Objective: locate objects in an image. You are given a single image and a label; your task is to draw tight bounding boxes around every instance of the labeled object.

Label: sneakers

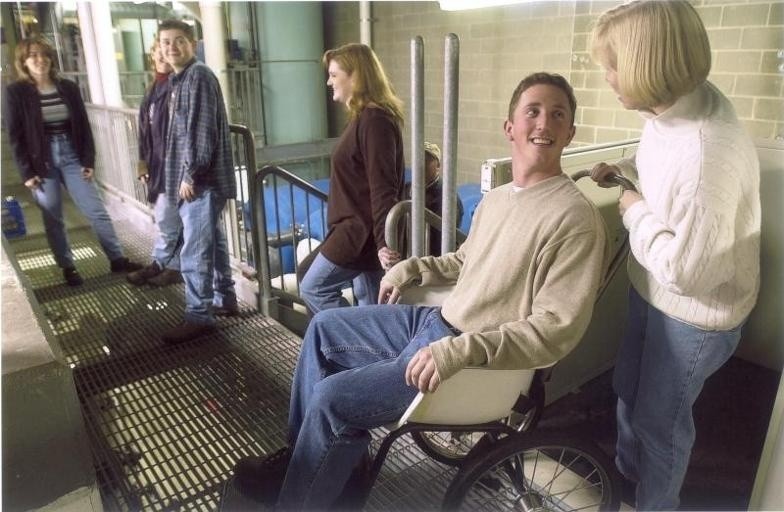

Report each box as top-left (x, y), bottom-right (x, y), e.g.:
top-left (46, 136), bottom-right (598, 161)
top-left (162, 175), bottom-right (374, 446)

top-left (166, 307), bottom-right (233, 343)
top-left (110, 257), bottom-right (179, 286)
top-left (63, 268), bottom-right (83, 286)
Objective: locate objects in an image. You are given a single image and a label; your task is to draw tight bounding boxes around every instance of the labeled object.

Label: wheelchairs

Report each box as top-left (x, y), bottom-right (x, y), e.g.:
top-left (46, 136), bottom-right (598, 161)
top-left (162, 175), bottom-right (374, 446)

top-left (215, 169), bottom-right (638, 512)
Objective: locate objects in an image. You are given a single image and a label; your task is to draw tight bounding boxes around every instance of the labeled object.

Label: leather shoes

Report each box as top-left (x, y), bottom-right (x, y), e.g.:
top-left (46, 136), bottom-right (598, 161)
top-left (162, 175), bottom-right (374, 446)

top-left (232, 446), bottom-right (293, 486)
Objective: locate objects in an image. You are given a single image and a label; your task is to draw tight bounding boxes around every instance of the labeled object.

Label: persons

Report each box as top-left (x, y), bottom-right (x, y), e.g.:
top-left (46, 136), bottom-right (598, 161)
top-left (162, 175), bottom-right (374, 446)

top-left (577, 1), bottom-right (763, 509)
top-left (406, 142), bottom-right (465, 253)
top-left (295, 40), bottom-right (404, 316)
top-left (150, 13), bottom-right (241, 347)
top-left (232, 72), bottom-right (611, 509)
top-left (5, 35), bottom-right (148, 288)
top-left (124, 38), bottom-right (187, 286)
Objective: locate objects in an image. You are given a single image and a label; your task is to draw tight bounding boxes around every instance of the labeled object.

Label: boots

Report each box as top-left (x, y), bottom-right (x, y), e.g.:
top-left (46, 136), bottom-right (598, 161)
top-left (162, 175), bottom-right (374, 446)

top-left (425, 142), bottom-right (440, 161)
